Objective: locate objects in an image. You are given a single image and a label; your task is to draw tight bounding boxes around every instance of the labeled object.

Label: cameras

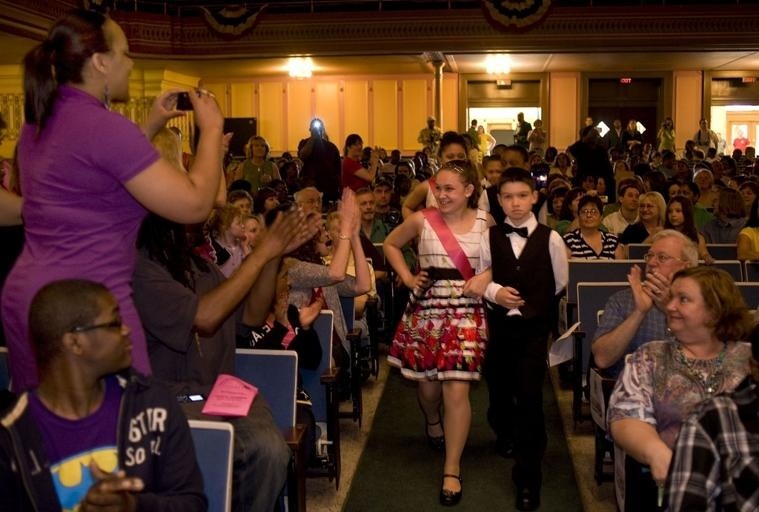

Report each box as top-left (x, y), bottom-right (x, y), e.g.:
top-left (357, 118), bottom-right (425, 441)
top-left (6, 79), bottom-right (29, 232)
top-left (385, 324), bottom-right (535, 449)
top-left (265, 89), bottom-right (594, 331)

top-left (177, 92), bottom-right (194, 110)
top-left (383, 209), bottom-right (401, 225)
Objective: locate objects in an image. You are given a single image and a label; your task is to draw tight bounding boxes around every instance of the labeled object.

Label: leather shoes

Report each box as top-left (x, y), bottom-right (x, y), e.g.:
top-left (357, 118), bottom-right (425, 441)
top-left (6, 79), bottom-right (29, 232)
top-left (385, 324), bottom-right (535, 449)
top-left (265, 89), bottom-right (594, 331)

top-left (440, 474), bottom-right (463, 506)
top-left (426, 418), bottom-right (445, 449)
top-left (516, 490), bottom-right (540, 510)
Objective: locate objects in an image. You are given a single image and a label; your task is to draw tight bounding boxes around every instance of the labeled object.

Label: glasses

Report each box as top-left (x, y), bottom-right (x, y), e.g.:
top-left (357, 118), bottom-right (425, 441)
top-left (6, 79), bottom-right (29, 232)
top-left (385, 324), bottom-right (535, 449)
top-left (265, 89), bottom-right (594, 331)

top-left (644, 254), bottom-right (677, 262)
top-left (75, 318), bottom-right (122, 331)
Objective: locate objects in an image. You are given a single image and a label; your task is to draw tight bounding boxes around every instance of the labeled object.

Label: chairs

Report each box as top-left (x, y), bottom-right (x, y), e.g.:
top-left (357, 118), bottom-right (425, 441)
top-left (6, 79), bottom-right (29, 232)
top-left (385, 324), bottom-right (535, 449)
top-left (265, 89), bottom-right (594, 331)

top-left (558, 242), bottom-right (759, 512)
top-left (1, 346), bottom-right (11, 391)
top-left (187, 238), bottom-right (387, 512)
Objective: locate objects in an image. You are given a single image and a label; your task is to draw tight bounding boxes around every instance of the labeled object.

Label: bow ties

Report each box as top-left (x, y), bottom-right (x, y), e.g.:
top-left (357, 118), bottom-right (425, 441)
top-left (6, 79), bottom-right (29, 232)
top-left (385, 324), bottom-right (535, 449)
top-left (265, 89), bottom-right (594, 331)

top-left (504, 223), bottom-right (528, 237)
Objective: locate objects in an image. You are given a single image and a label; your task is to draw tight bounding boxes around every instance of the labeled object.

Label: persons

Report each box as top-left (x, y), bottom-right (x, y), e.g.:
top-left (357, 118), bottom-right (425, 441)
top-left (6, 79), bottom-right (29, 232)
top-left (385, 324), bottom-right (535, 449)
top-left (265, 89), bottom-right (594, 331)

top-left (592, 229), bottom-right (699, 370)
top-left (467, 119), bottom-right (496, 154)
top-left (660, 322), bottom-right (759, 512)
top-left (515, 112), bottom-right (546, 157)
top-left (129, 200), bottom-right (321, 512)
top-left (382, 127), bottom-right (569, 512)
top-left (606, 263), bottom-right (759, 488)
top-left (0, 9), bottom-right (225, 392)
top-left (0, 277), bottom-right (209, 512)
top-left (562, 116), bottom-right (759, 263)
top-left (152, 118), bottom-right (442, 385)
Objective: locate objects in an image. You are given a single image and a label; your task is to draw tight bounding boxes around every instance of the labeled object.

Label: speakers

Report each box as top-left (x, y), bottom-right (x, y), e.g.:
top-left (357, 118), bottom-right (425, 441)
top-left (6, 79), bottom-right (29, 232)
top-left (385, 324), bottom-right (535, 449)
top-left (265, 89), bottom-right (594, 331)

top-left (194, 117), bottom-right (257, 158)
